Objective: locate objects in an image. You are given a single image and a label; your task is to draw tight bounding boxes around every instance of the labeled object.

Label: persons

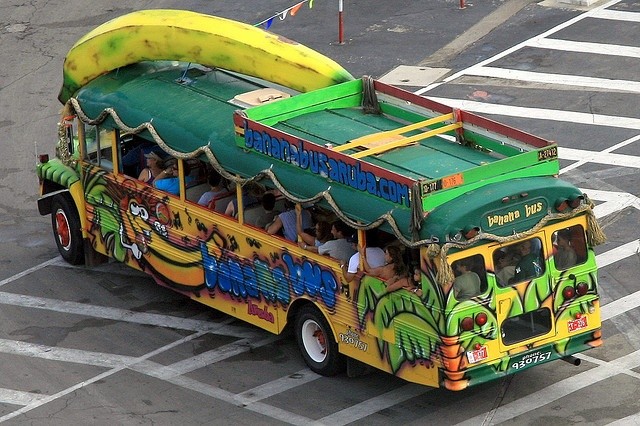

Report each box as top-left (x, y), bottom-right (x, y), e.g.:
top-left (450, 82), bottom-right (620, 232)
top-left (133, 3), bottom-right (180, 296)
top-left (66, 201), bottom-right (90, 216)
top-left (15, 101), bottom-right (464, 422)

top-left (357, 242), bottom-right (402, 281)
top-left (386, 263), bottom-right (413, 292)
top-left (255, 193), bottom-right (282, 228)
top-left (152, 158), bottom-right (195, 196)
top-left (297, 222), bottom-right (356, 264)
top-left (224, 184), bottom-right (259, 218)
top-left (266, 197), bottom-right (312, 242)
top-left (295, 206), bottom-right (334, 247)
top-left (121, 139), bottom-right (150, 178)
top-left (553, 229), bottom-right (578, 270)
top-left (412, 266), bottom-right (423, 297)
top-left (515, 241), bottom-right (544, 281)
top-left (340, 234), bottom-right (388, 283)
top-left (136, 150), bottom-right (164, 185)
top-left (196, 171), bottom-right (228, 206)
top-left (454, 259), bottom-right (482, 297)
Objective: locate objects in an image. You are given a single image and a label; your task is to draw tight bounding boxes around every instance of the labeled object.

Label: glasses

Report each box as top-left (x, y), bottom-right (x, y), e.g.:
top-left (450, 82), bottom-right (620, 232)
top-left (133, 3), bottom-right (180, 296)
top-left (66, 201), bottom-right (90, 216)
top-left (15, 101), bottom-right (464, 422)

top-left (173, 166), bottom-right (177, 170)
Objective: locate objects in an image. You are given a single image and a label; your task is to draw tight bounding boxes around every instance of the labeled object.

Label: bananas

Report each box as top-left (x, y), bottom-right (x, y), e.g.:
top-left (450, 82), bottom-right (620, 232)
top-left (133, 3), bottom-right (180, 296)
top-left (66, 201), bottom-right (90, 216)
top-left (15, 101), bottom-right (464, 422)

top-left (119, 194), bottom-right (224, 287)
top-left (59, 8), bottom-right (354, 106)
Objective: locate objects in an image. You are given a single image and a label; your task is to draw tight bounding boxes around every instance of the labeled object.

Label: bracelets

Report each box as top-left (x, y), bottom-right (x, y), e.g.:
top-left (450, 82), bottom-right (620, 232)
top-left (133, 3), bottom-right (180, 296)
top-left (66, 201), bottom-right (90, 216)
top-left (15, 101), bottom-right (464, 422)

top-left (162, 169), bottom-right (168, 176)
top-left (413, 289), bottom-right (417, 294)
top-left (341, 264), bottom-right (345, 268)
top-left (303, 244), bottom-right (307, 250)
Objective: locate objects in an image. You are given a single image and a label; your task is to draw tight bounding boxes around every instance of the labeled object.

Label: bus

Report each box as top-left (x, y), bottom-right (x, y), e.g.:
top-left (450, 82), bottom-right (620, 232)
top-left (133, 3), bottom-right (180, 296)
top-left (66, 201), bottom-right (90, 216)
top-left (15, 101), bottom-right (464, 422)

top-left (36, 8), bottom-right (609, 393)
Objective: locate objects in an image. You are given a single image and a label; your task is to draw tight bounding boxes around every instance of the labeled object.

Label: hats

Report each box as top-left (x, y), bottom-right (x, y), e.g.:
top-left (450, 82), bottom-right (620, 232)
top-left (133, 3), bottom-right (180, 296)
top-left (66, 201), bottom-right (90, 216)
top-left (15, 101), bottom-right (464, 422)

top-left (347, 233), bottom-right (358, 242)
top-left (144, 150), bottom-right (163, 161)
